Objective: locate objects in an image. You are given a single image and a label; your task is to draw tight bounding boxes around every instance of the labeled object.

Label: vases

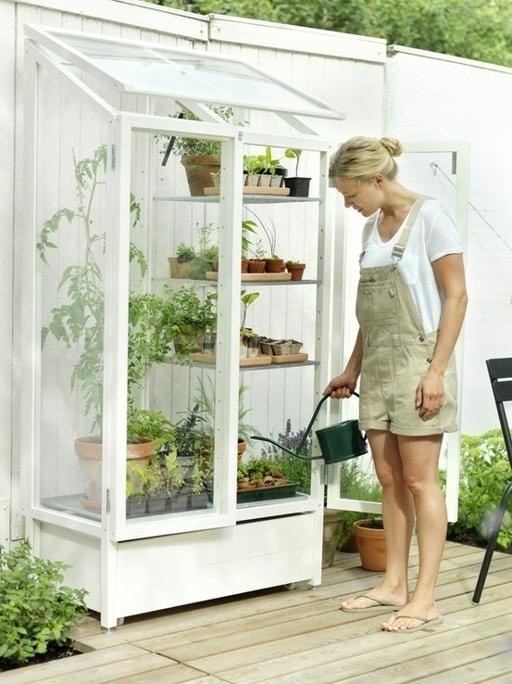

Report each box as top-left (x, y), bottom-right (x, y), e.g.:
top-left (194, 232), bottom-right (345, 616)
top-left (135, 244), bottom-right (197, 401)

top-left (354, 518), bottom-right (388, 572)
top-left (323, 505), bottom-right (348, 568)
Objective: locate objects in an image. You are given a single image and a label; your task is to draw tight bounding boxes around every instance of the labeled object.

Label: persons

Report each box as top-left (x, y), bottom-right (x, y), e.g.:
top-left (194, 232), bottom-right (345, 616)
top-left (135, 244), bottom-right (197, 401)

top-left (321, 135), bottom-right (469, 634)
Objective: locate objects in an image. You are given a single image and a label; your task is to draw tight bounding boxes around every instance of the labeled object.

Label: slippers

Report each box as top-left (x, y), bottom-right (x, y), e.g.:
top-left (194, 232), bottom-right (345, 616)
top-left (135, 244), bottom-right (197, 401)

top-left (382, 605), bottom-right (444, 632)
top-left (342, 591), bottom-right (409, 612)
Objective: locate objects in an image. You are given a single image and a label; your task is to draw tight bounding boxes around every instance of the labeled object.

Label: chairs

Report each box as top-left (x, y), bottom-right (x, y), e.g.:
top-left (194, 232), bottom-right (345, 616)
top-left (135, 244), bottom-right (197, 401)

top-left (475, 354), bottom-right (512, 602)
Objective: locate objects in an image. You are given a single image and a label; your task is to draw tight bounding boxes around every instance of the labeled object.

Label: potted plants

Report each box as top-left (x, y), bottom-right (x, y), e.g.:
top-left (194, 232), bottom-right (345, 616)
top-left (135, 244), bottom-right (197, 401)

top-left (36, 103), bottom-right (312, 517)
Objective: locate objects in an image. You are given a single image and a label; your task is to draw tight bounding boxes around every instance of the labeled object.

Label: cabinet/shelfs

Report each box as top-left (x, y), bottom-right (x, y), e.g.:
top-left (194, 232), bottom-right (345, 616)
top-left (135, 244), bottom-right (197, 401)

top-left (22, 23), bottom-right (467, 629)
